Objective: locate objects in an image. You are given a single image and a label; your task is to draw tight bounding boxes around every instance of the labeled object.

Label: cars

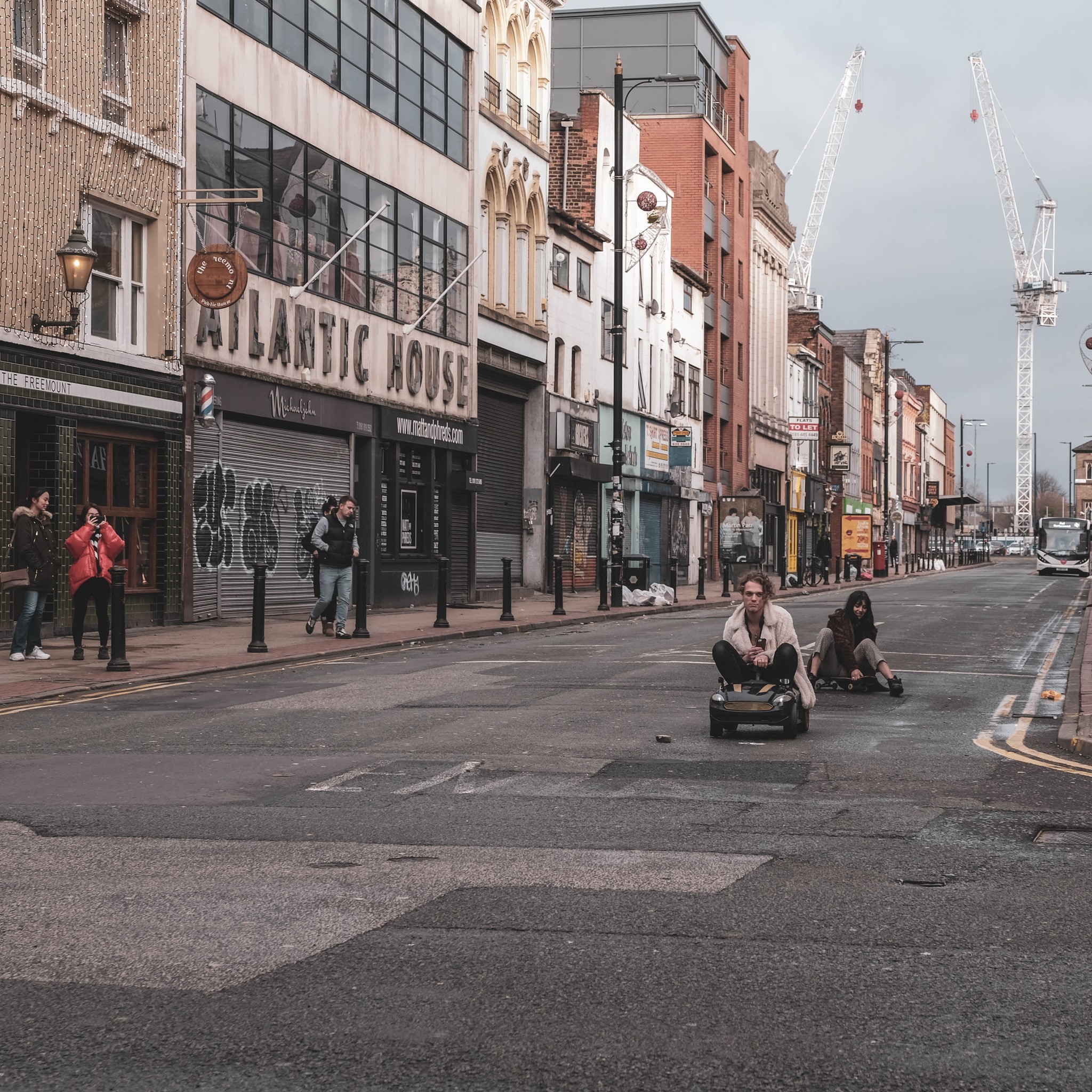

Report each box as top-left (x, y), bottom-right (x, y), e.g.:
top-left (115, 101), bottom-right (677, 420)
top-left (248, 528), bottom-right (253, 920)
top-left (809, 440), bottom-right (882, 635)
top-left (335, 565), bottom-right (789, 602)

top-left (709, 674), bottom-right (810, 740)
top-left (975, 542), bottom-right (994, 556)
top-left (989, 541), bottom-right (1006, 556)
top-left (1007, 542), bottom-right (1028, 556)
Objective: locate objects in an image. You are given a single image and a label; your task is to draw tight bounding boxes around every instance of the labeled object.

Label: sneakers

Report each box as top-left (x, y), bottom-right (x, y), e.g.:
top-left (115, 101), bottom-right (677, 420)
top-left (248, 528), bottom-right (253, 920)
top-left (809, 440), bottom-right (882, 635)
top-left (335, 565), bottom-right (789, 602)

top-left (306, 615), bottom-right (317, 635)
top-left (9, 652), bottom-right (24, 661)
top-left (73, 647), bottom-right (84, 661)
top-left (25, 645), bottom-right (51, 660)
top-left (335, 627), bottom-right (352, 639)
top-left (98, 647), bottom-right (110, 659)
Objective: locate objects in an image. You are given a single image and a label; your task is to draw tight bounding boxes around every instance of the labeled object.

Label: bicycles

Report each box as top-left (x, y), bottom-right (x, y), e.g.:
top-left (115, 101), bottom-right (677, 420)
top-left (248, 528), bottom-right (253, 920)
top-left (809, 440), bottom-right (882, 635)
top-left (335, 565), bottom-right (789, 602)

top-left (801, 554), bottom-right (824, 587)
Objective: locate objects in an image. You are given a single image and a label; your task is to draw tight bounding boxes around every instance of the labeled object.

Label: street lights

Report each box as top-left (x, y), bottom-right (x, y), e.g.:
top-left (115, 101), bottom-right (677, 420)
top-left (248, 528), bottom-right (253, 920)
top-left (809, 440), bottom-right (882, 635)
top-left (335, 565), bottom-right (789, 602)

top-left (987, 463), bottom-right (996, 520)
top-left (882, 332), bottom-right (925, 578)
top-left (964, 422), bottom-right (988, 548)
top-left (960, 412), bottom-right (985, 565)
top-left (1059, 442), bottom-right (1072, 518)
top-left (609, 52), bottom-right (701, 609)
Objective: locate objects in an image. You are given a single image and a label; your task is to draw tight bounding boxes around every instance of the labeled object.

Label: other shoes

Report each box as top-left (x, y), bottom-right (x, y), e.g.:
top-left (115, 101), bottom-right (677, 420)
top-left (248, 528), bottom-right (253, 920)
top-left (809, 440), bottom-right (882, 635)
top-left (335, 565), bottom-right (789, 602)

top-left (808, 673), bottom-right (818, 690)
top-left (321, 617), bottom-right (334, 637)
top-left (887, 675), bottom-right (904, 695)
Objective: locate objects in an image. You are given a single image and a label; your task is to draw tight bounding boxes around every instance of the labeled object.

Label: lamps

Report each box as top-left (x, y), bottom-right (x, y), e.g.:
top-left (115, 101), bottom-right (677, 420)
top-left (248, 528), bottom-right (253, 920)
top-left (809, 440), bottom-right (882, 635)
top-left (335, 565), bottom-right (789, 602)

top-left (30, 201), bottom-right (99, 334)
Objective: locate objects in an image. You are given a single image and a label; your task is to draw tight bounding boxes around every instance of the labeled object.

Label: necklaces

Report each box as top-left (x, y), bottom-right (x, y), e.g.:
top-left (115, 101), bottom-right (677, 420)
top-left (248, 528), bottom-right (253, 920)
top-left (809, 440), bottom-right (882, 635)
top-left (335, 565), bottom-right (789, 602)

top-left (745, 612), bottom-right (763, 639)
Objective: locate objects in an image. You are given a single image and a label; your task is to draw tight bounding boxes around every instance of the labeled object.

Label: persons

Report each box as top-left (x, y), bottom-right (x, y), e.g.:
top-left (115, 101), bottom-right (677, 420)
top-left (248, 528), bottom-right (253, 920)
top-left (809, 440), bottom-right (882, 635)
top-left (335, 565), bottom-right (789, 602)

top-left (720, 508), bottom-right (742, 561)
top-left (805, 590), bottom-right (905, 696)
top-left (302, 495), bottom-right (339, 636)
top-left (741, 509), bottom-right (763, 561)
top-left (64, 502), bottom-right (125, 661)
top-left (815, 532), bottom-right (833, 585)
top-left (888, 535), bottom-right (899, 568)
top-left (711, 571), bottom-right (817, 710)
top-left (304, 495), bottom-right (361, 641)
top-left (8, 488), bottom-right (62, 662)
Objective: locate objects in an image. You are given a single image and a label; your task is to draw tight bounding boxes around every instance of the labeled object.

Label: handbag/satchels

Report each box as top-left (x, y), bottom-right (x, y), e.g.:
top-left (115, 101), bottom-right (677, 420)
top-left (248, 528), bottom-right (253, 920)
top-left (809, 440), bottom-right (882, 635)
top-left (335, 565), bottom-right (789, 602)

top-left (1, 569), bottom-right (30, 590)
top-left (622, 582), bottom-right (675, 606)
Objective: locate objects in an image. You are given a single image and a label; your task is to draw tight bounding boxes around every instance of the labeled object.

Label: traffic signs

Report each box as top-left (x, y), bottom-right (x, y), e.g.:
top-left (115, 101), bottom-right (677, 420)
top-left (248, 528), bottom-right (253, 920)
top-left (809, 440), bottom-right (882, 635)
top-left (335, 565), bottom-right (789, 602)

top-left (840, 513), bottom-right (873, 560)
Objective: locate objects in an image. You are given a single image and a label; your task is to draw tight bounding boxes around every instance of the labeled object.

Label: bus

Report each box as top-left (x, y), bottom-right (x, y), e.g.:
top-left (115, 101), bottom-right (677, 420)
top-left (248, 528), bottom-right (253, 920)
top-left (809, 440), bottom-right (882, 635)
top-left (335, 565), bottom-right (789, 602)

top-left (1034, 517), bottom-right (1092, 578)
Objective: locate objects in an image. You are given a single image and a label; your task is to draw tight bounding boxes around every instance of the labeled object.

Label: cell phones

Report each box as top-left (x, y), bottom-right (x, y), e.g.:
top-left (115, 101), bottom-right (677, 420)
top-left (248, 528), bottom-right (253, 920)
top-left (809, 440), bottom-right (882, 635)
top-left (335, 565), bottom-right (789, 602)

top-left (756, 638), bottom-right (766, 651)
top-left (90, 516), bottom-right (105, 523)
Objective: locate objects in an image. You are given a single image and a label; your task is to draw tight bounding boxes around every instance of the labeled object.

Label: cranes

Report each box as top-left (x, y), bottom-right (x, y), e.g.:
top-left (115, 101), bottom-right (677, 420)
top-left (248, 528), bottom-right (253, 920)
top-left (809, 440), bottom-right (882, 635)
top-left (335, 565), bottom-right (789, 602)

top-left (778, 42), bottom-right (868, 313)
top-left (964, 51), bottom-right (1069, 531)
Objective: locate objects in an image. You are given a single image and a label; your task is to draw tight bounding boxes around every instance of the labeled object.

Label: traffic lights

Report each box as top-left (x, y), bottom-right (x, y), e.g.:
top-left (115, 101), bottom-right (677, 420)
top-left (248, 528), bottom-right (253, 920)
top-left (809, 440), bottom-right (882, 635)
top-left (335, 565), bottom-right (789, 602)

top-left (952, 539), bottom-right (955, 543)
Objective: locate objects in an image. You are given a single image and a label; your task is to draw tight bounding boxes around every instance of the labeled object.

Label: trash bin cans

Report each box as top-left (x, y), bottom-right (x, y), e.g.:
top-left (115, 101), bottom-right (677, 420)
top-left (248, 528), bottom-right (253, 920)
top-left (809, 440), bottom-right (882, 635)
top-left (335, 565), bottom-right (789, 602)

top-left (622, 553), bottom-right (651, 592)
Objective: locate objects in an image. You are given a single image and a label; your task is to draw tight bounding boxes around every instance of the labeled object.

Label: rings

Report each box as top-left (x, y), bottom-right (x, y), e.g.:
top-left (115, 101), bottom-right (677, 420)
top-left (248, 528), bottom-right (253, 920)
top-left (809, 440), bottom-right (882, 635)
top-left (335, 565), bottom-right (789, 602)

top-left (853, 677), bottom-right (855, 679)
top-left (757, 659), bottom-right (762, 662)
